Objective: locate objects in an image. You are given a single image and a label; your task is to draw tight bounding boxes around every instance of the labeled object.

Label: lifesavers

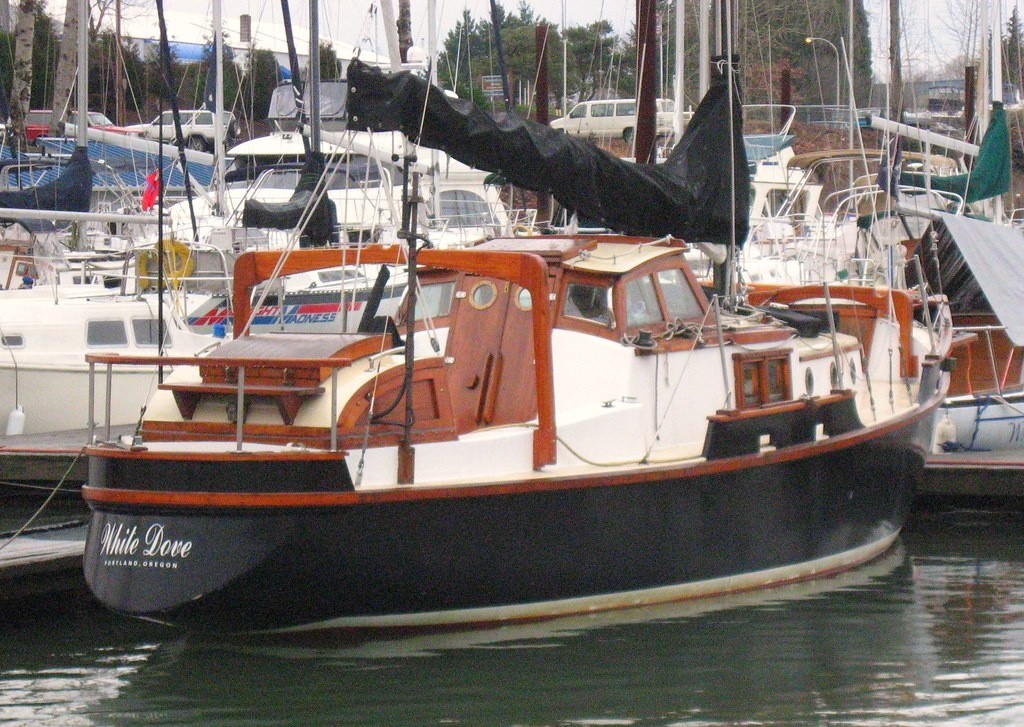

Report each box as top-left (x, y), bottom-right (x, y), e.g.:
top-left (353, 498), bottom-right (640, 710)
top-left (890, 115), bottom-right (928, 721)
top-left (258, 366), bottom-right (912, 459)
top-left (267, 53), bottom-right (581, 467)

top-left (137, 249), bottom-right (171, 288)
top-left (155, 238), bottom-right (195, 278)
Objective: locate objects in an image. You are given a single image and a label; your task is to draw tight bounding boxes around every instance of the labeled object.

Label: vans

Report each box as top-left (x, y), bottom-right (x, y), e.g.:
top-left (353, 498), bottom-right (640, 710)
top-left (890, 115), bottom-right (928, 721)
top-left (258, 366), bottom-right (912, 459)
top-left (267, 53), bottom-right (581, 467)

top-left (683, 111), bottom-right (696, 126)
top-left (547, 97), bottom-right (677, 142)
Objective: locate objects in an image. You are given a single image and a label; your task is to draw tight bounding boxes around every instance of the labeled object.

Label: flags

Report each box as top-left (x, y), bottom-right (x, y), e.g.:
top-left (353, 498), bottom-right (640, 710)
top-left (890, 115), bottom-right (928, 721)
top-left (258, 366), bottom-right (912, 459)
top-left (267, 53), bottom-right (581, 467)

top-left (874, 138), bottom-right (903, 199)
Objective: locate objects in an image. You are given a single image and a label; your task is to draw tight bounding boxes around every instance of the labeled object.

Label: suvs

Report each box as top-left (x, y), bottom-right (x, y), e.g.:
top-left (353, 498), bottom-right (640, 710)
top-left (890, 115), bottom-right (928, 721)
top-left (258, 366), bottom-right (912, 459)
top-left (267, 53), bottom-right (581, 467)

top-left (120, 110), bottom-right (243, 155)
top-left (22, 110), bottom-right (148, 147)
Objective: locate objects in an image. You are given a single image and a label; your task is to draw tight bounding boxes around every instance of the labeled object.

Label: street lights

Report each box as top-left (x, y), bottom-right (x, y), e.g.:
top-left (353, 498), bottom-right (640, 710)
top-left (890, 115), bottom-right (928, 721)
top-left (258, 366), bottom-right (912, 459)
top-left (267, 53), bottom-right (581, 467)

top-left (805, 37), bottom-right (841, 120)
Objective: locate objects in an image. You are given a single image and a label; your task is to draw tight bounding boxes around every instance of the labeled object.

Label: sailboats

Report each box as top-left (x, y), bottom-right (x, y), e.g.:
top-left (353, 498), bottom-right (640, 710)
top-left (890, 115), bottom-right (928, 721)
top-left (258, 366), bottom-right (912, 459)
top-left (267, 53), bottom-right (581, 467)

top-left (0, 0), bottom-right (1024, 644)
